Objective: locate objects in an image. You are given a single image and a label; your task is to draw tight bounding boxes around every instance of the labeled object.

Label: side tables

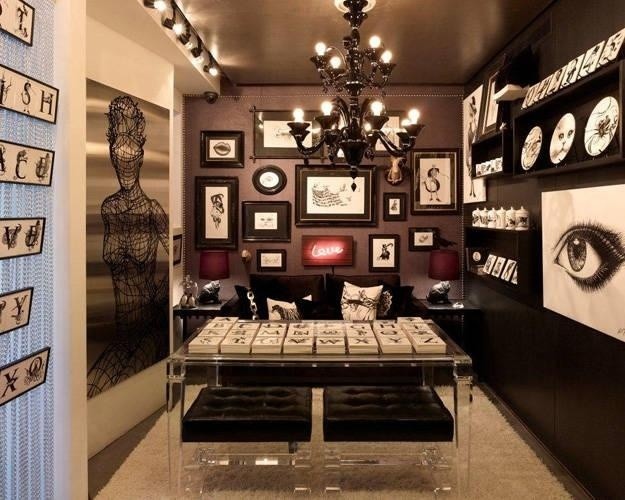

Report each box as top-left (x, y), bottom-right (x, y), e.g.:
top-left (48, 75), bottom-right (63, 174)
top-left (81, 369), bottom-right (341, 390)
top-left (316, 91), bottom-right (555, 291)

top-left (416, 299), bottom-right (479, 354)
top-left (173, 299), bottom-right (228, 340)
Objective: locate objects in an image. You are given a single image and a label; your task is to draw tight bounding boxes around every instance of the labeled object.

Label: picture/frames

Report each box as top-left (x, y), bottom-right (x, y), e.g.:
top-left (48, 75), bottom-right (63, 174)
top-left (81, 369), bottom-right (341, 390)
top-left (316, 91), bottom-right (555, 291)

top-left (172, 234), bottom-right (183, 265)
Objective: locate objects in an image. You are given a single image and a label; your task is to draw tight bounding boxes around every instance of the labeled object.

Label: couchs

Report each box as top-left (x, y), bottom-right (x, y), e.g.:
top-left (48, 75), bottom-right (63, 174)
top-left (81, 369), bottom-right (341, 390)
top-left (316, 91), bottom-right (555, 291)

top-left (220, 271), bottom-right (429, 388)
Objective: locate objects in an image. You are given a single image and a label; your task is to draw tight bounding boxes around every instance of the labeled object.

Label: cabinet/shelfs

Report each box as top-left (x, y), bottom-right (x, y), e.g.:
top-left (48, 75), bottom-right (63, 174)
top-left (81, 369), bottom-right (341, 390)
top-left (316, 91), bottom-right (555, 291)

top-left (471, 129), bottom-right (513, 178)
top-left (511, 60), bottom-right (625, 180)
top-left (463, 224), bottom-right (537, 307)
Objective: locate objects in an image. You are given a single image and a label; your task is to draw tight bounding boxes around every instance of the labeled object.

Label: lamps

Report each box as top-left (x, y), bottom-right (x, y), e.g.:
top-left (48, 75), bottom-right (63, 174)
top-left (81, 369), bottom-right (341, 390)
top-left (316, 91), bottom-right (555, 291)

top-left (144, 0), bottom-right (222, 78)
top-left (286, 0), bottom-right (426, 194)
top-left (475, 51), bottom-right (511, 141)
top-left (429, 249), bottom-right (461, 282)
top-left (198, 249), bottom-right (231, 280)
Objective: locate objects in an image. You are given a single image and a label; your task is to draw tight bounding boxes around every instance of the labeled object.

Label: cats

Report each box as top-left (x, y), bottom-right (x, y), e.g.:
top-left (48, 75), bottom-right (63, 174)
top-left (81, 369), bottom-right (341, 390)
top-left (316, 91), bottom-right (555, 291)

top-left (549, 113), bottom-right (575, 164)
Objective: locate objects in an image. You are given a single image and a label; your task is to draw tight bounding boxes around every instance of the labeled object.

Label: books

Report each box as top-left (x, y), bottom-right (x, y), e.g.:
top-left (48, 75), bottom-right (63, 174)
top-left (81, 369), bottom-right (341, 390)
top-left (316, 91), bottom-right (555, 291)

top-left (188, 314), bottom-right (446, 354)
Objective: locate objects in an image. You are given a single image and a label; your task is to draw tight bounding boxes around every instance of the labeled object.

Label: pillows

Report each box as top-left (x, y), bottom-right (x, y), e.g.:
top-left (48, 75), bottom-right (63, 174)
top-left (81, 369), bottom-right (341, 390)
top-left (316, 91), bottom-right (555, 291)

top-left (234, 284), bottom-right (315, 322)
top-left (377, 280), bottom-right (415, 320)
top-left (340, 280), bottom-right (383, 321)
